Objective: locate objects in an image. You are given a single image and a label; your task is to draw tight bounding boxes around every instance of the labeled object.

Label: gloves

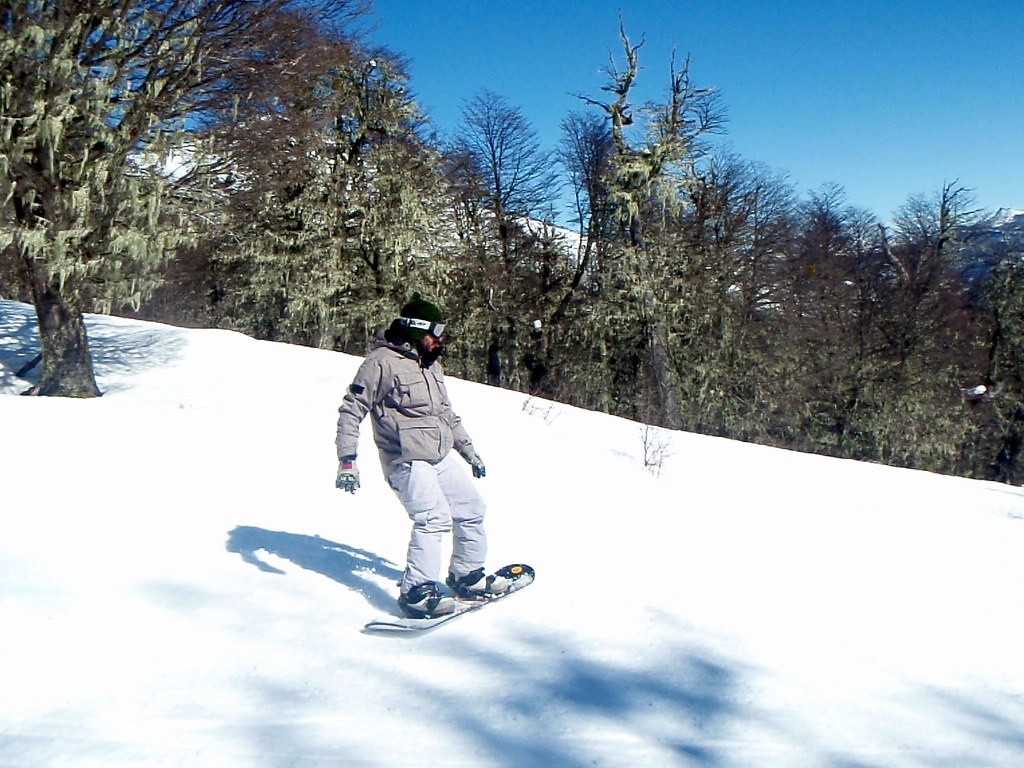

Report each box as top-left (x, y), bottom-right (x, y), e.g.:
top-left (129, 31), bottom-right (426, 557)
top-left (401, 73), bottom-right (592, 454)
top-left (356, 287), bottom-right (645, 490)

top-left (336, 455), bottom-right (360, 494)
top-left (459, 445), bottom-right (485, 478)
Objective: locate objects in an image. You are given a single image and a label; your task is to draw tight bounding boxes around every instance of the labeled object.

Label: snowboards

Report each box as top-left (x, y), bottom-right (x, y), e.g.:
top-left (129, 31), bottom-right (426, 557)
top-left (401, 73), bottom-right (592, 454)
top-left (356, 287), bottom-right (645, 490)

top-left (365, 563), bottom-right (535, 631)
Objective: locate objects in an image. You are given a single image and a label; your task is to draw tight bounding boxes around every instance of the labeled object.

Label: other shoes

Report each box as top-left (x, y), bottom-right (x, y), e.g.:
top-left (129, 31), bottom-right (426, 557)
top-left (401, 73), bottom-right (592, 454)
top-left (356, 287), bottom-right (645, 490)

top-left (397, 581), bottom-right (455, 618)
top-left (446, 566), bottom-right (512, 598)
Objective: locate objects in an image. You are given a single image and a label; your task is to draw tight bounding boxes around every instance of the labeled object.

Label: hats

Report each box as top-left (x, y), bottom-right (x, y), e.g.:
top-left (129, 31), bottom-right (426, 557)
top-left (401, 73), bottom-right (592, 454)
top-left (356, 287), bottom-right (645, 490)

top-left (385, 292), bottom-right (446, 369)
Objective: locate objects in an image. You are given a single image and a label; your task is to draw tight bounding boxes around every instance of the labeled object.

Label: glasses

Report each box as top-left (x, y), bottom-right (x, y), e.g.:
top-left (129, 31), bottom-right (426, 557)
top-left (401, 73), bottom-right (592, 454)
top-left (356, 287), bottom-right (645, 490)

top-left (400, 318), bottom-right (445, 339)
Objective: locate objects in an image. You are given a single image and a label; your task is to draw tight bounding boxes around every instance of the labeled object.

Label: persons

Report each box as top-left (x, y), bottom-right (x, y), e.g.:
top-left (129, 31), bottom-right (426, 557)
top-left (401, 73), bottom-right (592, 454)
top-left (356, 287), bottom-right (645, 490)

top-left (335, 293), bottom-right (507, 614)
